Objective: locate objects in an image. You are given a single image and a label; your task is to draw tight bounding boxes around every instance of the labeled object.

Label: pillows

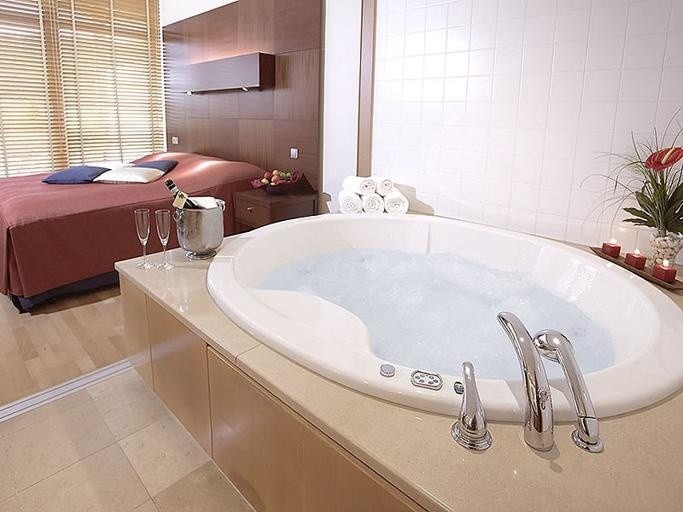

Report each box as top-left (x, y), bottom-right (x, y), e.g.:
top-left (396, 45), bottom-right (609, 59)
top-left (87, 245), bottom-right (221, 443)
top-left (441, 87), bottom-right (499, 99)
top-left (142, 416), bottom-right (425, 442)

top-left (92, 166), bottom-right (165, 183)
top-left (133, 160), bottom-right (179, 173)
top-left (42, 166), bottom-right (110, 184)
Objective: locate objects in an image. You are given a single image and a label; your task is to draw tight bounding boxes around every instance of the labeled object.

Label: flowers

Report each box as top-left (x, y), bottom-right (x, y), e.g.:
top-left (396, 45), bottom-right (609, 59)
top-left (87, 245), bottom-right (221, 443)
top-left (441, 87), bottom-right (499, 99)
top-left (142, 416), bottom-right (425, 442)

top-left (580, 108), bottom-right (683, 235)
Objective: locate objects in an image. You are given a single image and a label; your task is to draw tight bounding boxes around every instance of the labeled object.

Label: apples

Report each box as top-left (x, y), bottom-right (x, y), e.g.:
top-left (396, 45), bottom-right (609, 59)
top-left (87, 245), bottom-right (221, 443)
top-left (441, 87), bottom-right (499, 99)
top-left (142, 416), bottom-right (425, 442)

top-left (261, 170), bottom-right (294, 185)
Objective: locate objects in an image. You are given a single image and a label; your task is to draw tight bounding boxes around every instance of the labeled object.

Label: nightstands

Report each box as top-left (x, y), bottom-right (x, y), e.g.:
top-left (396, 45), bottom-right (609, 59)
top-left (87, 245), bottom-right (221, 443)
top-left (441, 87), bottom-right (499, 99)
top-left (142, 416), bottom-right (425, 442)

top-left (234, 189), bottom-right (319, 234)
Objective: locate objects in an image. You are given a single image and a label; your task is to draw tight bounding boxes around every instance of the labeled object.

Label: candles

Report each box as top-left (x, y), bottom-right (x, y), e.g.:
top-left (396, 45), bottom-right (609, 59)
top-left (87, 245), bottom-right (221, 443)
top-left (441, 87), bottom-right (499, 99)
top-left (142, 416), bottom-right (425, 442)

top-left (653, 258), bottom-right (677, 282)
top-left (625, 248), bottom-right (647, 269)
top-left (602, 238), bottom-right (621, 257)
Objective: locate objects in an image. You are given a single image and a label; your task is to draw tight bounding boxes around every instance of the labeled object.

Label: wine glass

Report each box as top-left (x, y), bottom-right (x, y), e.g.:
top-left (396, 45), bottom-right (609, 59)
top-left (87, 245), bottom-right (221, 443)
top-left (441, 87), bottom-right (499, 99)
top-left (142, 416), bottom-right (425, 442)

top-left (153, 209), bottom-right (174, 272)
top-left (133, 207), bottom-right (151, 268)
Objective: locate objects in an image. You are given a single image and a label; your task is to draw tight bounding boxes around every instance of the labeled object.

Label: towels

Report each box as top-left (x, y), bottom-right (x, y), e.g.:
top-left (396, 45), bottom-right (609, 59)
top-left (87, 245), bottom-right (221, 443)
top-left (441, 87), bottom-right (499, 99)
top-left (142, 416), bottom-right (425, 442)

top-left (341, 174), bottom-right (376, 197)
top-left (363, 193), bottom-right (386, 216)
top-left (384, 186), bottom-right (410, 216)
top-left (337, 189), bottom-right (366, 215)
top-left (368, 173), bottom-right (394, 197)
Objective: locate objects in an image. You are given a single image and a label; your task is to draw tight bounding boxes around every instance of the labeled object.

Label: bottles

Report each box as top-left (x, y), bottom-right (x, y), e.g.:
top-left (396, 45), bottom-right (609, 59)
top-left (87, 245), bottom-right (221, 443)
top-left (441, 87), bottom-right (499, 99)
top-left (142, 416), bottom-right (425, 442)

top-left (165, 179), bottom-right (198, 209)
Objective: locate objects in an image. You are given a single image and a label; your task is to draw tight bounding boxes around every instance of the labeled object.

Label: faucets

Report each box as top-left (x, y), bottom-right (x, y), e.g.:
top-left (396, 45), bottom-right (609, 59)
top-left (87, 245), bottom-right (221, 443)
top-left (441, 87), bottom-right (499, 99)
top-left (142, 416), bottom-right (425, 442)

top-left (484, 310), bottom-right (556, 453)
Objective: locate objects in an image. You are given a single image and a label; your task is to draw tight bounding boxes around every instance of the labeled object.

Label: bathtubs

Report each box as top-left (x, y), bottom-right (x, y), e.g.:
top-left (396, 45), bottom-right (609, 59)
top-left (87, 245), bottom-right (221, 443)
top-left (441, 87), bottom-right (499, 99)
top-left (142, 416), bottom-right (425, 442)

top-left (205, 213), bottom-right (683, 461)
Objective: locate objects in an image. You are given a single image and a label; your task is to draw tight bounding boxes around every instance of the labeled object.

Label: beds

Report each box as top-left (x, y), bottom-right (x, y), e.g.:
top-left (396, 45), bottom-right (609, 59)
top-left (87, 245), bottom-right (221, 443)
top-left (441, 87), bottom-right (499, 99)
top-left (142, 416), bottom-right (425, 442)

top-left (3, 149), bottom-right (270, 316)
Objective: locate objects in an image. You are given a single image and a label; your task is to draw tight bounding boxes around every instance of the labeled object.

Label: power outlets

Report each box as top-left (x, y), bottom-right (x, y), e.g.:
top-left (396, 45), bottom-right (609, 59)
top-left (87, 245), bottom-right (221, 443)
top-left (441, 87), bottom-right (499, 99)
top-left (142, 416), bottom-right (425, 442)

top-left (171, 137), bottom-right (179, 144)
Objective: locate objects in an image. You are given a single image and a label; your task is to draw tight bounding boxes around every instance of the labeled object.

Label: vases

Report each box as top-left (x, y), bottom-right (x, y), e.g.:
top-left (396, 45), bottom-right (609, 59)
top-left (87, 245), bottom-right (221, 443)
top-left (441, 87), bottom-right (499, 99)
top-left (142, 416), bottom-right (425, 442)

top-left (649, 232), bottom-right (683, 268)
